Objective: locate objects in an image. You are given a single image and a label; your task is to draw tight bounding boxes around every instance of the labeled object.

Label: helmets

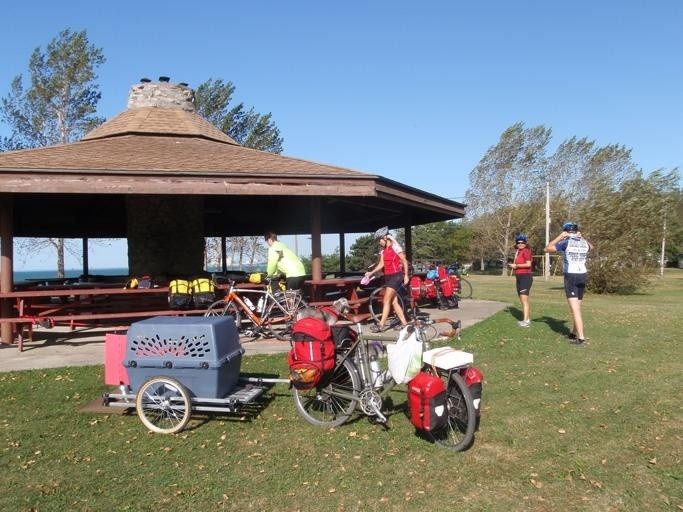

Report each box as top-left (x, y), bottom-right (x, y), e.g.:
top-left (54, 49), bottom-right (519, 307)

top-left (374, 226), bottom-right (388, 241)
top-left (562, 222), bottom-right (578, 232)
top-left (516, 234), bottom-right (527, 241)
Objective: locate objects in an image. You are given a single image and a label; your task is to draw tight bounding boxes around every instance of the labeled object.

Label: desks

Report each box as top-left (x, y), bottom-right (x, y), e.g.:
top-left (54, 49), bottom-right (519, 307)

top-left (0, 281), bottom-right (266, 352)
top-left (301, 276), bottom-right (378, 314)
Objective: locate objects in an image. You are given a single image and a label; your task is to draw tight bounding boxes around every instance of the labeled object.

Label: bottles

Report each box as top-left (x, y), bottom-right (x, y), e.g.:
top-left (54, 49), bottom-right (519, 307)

top-left (256, 296), bottom-right (265, 313)
top-left (242, 296), bottom-right (257, 312)
top-left (369, 354), bottom-right (384, 391)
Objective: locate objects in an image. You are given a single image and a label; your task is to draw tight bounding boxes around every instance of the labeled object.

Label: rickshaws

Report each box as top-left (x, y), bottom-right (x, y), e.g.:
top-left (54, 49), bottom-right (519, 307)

top-left (100, 375), bottom-right (269, 436)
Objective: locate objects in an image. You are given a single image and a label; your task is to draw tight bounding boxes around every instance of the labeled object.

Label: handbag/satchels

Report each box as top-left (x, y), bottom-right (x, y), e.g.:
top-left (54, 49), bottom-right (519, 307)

top-left (449, 369), bottom-right (482, 434)
top-left (409, 266), bottom-right (460, 302)
top-left (408, 373), bottom-right (446, 432)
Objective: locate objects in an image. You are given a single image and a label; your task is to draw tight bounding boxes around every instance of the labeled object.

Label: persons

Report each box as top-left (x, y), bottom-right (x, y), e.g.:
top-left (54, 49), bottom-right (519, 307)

top-left (363, 226), bottom-right (410, 334)
top-left (507, 234), bottom-right (534, 329)
top-left (264, 230), bottom-right (307, 340)
top-left (542, 221), bottom-right (595, 344)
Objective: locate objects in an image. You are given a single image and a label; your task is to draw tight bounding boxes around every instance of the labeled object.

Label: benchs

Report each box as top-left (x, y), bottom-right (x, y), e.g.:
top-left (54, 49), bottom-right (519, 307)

top-left (308, 296), bottom-right (377, 313)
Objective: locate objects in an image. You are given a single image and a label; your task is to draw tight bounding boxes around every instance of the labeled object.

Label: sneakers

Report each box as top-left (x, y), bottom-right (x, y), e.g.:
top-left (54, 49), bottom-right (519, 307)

top-left (394, 323), bottom-right (406, 330)
top-left (566, 334), bottom-right (588, 346)
top-left (370, 325), bottom-right (383, 331)
top-left (519, 321), bottom-right (532, 328)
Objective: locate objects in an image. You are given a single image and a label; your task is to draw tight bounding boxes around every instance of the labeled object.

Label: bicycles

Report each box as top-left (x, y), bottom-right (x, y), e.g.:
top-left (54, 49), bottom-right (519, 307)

top-left (202, 272), bottom-right (313, 343)
top-left (432, 261), bottom-right (473, 309)
top-left (289, 281), bottom-right (478, 452)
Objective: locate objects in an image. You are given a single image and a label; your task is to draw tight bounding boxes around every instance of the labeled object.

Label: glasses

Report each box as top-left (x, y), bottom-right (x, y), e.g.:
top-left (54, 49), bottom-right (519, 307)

top-left (516, 242), bottom-right (526, 244)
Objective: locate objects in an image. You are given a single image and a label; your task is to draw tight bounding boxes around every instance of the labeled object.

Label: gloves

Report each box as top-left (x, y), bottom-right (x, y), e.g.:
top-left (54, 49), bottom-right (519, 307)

top-left (263, 277), bottom-right (271, 284)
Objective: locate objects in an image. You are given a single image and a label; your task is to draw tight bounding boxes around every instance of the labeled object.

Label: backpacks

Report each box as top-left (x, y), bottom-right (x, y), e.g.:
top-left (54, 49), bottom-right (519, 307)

top-left (167, 279), bottom-right (193, 310)
top-left (124, 273), bottom-right (154, 290)
top-left (249, 273), bottom-right (267, 285)
top-left (286, 317), bottom-right (335, 387)
top-left (193, 278), bottom-right (215, 309)
